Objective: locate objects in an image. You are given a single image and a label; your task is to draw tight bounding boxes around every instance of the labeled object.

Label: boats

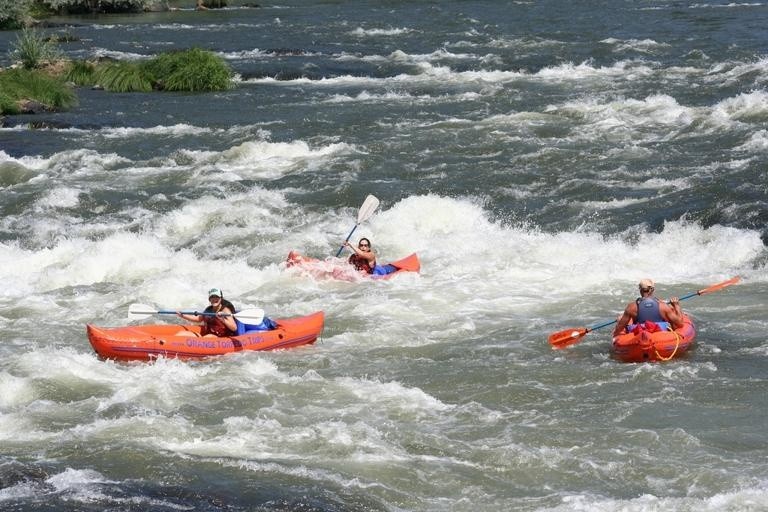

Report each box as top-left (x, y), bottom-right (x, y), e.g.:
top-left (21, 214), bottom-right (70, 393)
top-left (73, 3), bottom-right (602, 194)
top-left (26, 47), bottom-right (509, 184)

top-left (609, 308), bottom-right (698, 364)
top-left (286, 250), bottom-right (421, 286)
top-left (85, 309), bottom-right (327, 366)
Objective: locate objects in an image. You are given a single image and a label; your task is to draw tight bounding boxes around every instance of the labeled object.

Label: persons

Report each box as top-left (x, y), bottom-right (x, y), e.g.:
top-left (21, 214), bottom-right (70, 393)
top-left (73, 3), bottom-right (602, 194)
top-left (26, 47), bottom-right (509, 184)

top-left (613, 278), bottom-right (684, 337)
top-left (342, 238), bottom-right (376, 274)
top-left (175, 288), bottom-right (237, 338)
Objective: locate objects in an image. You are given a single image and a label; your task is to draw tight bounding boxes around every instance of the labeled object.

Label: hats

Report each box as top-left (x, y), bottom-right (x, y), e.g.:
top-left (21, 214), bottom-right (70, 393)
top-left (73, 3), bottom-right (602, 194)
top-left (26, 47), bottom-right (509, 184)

top-left (208, 288), bottom-right (223, 298)
top-left (638, 278), bottom-right (656, 290)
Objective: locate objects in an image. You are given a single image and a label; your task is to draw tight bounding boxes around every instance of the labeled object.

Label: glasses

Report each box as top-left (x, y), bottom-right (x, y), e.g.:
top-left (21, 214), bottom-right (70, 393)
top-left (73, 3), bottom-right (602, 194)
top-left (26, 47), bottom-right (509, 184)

top-left (359, 244), bottom-right (368, 247)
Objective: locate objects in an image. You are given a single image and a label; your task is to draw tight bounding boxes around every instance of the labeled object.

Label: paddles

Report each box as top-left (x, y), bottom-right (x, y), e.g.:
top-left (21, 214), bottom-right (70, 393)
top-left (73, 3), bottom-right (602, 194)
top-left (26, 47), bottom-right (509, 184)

top-left (548, 275), bottom-right (740, 348)
top-left (128, 304), bottom-right (264, 326)
top-left (335, 195), bottom-right (380, 257)
